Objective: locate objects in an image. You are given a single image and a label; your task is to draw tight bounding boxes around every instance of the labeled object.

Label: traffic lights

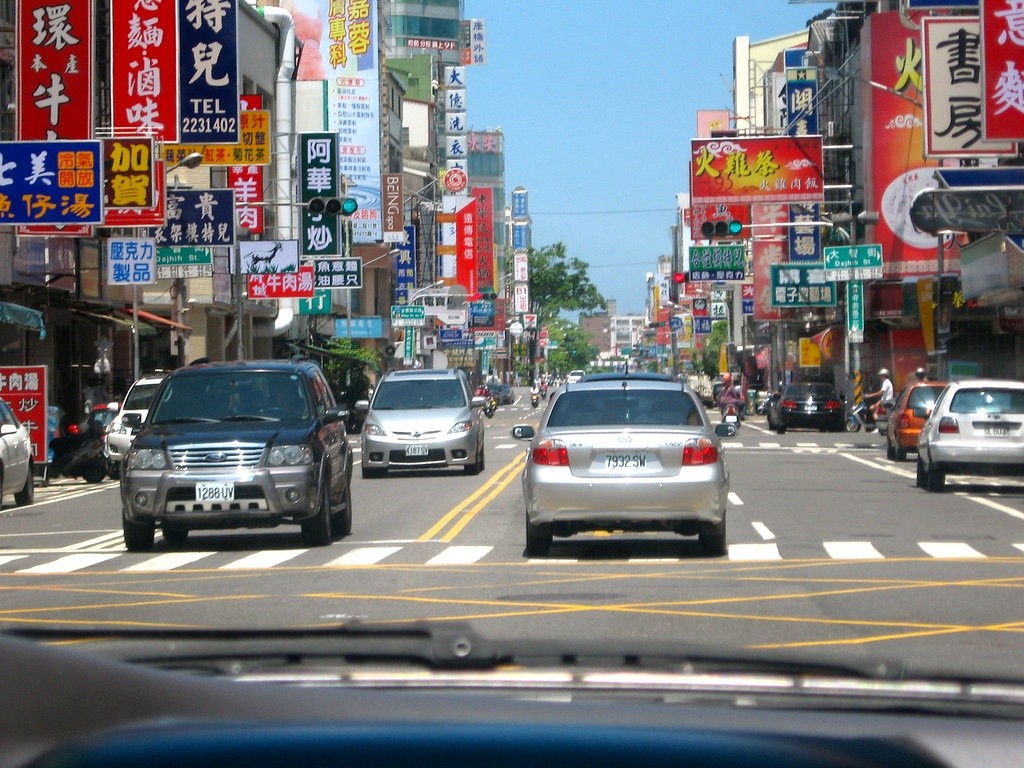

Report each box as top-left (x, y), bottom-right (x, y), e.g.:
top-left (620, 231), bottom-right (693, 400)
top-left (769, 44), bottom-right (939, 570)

top-left (674, 272), bottom-right (689, 283)
top-left (308, 196), bottom-right (358, 216)
top-left (473, 293), bottom-right (498, 300)
top-left (702, 220), bottom-right (743, 238)
top-left (649, 322), bottom-right (665, 328)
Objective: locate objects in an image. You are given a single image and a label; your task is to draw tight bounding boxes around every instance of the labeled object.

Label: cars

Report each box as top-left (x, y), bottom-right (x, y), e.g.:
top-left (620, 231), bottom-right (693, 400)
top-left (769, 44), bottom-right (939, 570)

top-left (511, 370), bottom-right (738, 560)
top-left (492, 384), bottom-right (515, 404)
top-left (0, 397), bottom-right (123, 511)
top-left (883, 380), bottom-right (1024, 492)
top-left (768, 382), bottom-right (844, 434)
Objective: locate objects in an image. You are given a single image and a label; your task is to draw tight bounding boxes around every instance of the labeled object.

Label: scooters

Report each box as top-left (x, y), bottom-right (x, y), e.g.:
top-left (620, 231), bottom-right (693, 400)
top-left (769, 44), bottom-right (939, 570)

top-left (844, 389), bottom-right (895, 433)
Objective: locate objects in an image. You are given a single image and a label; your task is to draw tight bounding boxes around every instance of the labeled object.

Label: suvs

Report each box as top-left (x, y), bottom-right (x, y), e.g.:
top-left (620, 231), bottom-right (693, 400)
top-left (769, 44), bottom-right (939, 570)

top-left (354, 367), bottom-right (487, 479)
top-left (105, 353), bottom-right (354, 553)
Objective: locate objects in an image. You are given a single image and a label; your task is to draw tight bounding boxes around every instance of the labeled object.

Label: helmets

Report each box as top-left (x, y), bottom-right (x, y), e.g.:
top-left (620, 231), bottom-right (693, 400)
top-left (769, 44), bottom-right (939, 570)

top-left (877, 368), bottom-right (889, 377)
top-left (722, 372), bottom-right (732, 381)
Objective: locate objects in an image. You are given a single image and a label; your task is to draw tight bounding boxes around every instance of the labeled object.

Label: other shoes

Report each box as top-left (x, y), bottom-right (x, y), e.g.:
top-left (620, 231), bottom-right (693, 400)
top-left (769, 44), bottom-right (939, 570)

top-left (871, 429), bottom-right (878, 433)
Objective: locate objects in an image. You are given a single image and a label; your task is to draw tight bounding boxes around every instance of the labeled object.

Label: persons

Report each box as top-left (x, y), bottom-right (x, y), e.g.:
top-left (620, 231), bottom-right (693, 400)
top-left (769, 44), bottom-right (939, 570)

top-left (863, 368), bottom-right (893, 433)
top-left (475, 385), bottom-right (497, 417)
top-left (717, 373), bottom-right (747, 422)
top-left (529, 373), bottom-right (564, 405)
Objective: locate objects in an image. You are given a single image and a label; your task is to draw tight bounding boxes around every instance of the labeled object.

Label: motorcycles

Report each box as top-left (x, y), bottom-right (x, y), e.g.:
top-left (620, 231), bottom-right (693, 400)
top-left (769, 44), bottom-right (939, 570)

top-left (721, 404), bottom-right (741, 429)
top-left (50, 411), bottom-right (109, 483)
top-left (483, 387), bottom-right (546, 418)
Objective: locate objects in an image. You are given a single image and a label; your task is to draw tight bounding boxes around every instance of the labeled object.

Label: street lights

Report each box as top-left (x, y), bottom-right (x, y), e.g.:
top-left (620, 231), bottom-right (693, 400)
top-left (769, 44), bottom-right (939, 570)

top-left (134, 153), bottom-right (203, 380)
top-left (823, 66), bottom-right (943, 328)
top-left (347, 248), bottom-right (402, 347)
top-left (666, 289), bottom-right (731, 373)
